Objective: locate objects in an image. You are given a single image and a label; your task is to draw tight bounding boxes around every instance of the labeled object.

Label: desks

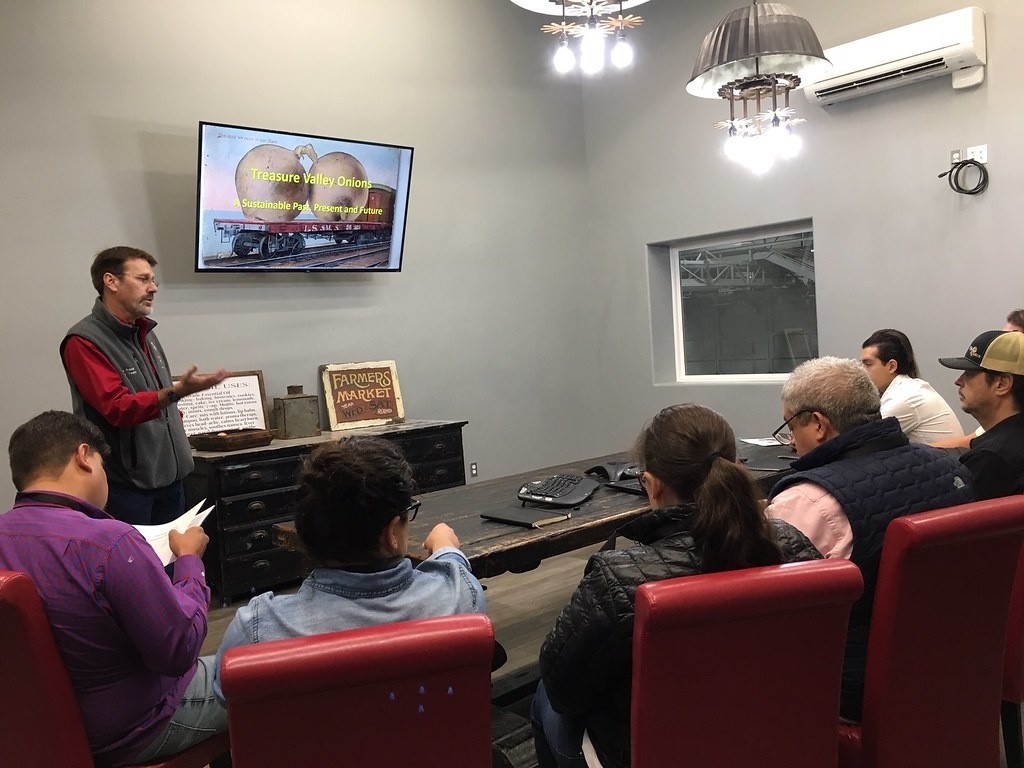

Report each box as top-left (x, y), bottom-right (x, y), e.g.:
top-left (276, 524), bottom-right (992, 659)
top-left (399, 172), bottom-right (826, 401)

top-left (272, 437), bottom-right (800, 581)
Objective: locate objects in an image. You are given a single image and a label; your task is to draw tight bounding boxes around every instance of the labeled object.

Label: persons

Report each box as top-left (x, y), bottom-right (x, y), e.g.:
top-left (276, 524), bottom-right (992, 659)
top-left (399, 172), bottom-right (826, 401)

top-left (208, 436), bottom-right (486, 712)
top-left (758, 356), bottom-right (978, 726)
top-left (58, 248), bottom-right (233, 585)
top-left (530, 403), bottom-right (827, 768)
top-left (861, 310), bottom-right (1024, 502)
top-left (0, 409), bottom-right (231, 768)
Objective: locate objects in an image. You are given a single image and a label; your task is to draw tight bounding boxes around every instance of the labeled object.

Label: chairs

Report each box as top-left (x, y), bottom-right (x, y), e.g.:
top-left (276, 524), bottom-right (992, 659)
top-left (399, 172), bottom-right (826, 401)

top-left (839, 494), bottom-right (1024, 768)
top-left (630, 559), bottom-right (865, 768)
top-left (220, 614), bottom-right (496, 767)
top-left (0, 568), bottom-right (231, 768)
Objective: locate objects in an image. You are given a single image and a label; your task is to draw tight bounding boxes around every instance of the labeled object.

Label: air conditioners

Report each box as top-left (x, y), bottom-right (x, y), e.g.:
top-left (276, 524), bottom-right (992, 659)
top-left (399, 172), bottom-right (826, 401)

top-left (803, 6), bottom-right (986, 105)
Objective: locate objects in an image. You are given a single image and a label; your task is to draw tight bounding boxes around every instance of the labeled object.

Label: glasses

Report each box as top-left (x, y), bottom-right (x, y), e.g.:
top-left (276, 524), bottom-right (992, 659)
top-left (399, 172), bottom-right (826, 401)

top-left (772, 408), bottom-right (830, 445)
top-left (399, 499), bottom-right (421, 522)
top-left (637, 469), bottom-right (653, 491)
top-left (113, 272), bottom-right (160, 287)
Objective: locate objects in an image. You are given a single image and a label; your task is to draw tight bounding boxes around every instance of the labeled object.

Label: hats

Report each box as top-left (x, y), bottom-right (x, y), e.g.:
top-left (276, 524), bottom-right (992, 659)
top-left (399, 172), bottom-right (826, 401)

top-left (938, 331), bottom-right (1024, 375)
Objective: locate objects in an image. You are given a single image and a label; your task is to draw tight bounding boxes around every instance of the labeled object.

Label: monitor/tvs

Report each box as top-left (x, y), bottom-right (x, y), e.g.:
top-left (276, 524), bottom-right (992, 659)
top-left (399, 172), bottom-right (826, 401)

top-left (194, 121), bottom-right (414, 272)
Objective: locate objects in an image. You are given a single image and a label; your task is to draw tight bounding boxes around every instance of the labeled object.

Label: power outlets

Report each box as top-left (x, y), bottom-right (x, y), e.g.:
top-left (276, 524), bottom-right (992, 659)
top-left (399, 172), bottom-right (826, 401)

top-left (951, 150), bottom-right (961, 167)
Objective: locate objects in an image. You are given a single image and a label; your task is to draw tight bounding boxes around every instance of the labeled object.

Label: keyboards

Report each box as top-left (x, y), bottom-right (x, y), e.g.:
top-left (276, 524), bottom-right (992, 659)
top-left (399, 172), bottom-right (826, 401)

top-left (517, 470), bottom-right (601, 509)
top-left (605, 478), bottom-right (645, 495)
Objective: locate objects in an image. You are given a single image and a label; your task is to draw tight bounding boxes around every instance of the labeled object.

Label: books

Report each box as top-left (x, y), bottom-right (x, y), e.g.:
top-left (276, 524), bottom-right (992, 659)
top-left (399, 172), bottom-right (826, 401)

top-left (739, 438), bottom-right (782, 447)
top-left (480, 504), bottom-right (568, 528)
top-left (129, 497), bottom-right (215, 568)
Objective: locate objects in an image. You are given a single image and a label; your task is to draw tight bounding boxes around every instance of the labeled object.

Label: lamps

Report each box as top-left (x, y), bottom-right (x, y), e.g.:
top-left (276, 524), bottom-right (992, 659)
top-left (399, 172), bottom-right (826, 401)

top-left (685, 0), bottom-right (834, 175)
top-left (510, 0), bottom-right (650, 76)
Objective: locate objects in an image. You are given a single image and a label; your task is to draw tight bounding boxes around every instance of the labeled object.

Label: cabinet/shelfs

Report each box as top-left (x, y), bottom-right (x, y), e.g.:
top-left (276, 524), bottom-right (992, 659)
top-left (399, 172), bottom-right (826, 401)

top-left (185, 420), bottom-right (469, 608)
